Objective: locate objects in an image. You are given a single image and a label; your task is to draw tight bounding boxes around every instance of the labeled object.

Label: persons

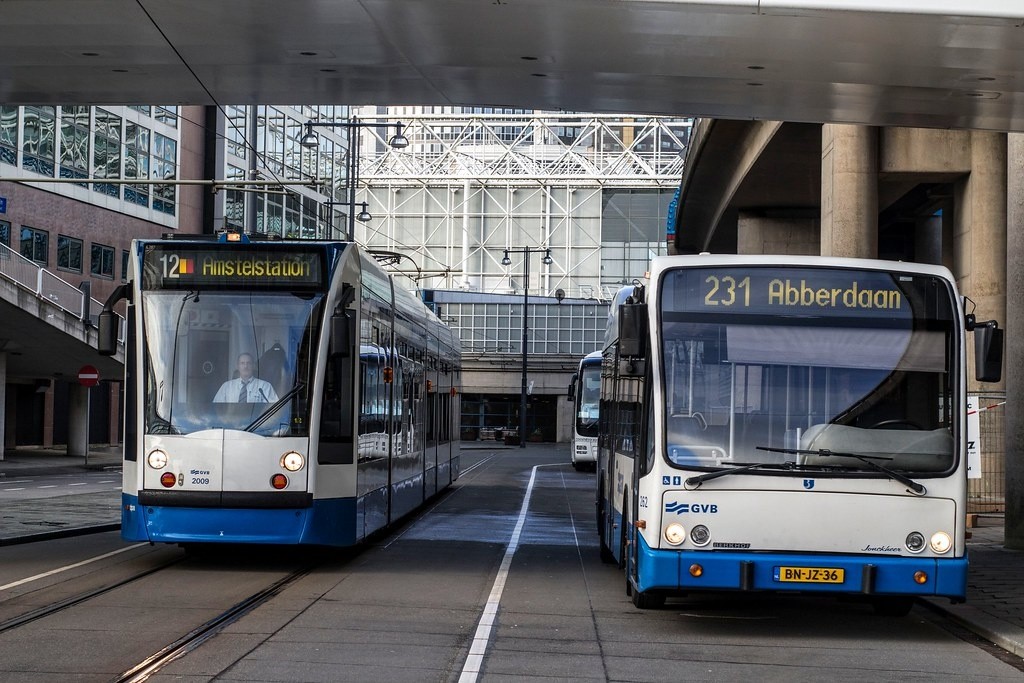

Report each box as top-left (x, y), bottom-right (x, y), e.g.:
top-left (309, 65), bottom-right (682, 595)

top-left (213, 352), bottom-right (279, 403)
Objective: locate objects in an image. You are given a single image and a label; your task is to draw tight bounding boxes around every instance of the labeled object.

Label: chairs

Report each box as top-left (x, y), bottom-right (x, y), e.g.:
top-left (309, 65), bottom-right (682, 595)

top-left (584, 388), bottom-right (600, 403)
top-left (234, 368), bottom-right (281, 391)
top-left (667, 415), bottom-right (704, 438)
top-left (728, 410), bottom-right (824, 452)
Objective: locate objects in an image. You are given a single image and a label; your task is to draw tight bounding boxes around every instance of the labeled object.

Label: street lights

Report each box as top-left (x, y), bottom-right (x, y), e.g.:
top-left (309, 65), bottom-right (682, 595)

top-left (500, 245), bottom-right (553, 447)
top-left (322, 198), bottom-right (373, 240)
top-left (299, 115), bottom-right (409, 242)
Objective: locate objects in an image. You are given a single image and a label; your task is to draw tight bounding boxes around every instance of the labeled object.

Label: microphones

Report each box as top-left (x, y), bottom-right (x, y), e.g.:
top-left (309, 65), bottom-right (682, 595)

top-left (259, 388), bottom-right (269, 402)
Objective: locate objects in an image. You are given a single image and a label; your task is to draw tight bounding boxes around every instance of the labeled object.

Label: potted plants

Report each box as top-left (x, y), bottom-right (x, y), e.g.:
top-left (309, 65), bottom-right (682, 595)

top-left (505, 432), bottom-right (521, 446)
top-left (461, 427), bottom-right (477, 440)
top-left (531, 428), bottom-right (543, 442)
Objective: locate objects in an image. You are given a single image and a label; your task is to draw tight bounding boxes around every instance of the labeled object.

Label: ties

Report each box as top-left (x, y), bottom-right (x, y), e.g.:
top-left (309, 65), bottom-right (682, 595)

top-left (239, 381), bottom-right (250, 403)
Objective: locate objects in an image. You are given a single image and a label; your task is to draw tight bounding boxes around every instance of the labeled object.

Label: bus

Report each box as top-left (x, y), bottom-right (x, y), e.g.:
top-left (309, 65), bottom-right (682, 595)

top-left (97, 223), bottom-right (460, 558)
top-left (595, 253), bottom-right (1003, 620)
top-left (567, 350), bottom-right (604, 473)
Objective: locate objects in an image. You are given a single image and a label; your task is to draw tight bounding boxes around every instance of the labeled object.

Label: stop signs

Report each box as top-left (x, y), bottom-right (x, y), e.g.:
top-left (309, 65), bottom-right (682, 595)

top-left (77, 365), bottom-right (99, 388)
